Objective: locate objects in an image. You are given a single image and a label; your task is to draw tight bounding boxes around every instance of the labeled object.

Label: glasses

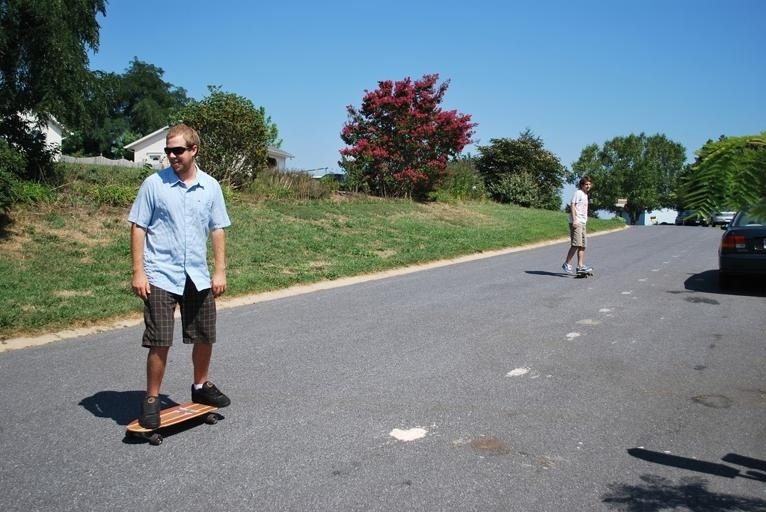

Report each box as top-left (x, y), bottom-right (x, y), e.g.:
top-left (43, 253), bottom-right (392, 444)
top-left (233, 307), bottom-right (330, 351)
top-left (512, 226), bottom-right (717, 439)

top-left (165, 146), bottom-right (190, 154)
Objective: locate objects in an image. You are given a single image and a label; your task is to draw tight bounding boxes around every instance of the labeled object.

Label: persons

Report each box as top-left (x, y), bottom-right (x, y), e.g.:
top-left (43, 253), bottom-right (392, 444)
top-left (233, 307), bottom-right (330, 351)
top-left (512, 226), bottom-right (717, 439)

top-left (562, 176), bottom-right (592, 274)
top-left (128, 124), bottom-right (231, 429)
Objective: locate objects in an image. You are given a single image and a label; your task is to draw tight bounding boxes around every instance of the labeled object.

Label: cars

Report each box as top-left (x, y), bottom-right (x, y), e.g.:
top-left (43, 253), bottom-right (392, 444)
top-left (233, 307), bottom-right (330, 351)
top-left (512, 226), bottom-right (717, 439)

top-left (676, 210), bottom-right (737, 227)
top-left (719, 201), bottom-right (766, 288)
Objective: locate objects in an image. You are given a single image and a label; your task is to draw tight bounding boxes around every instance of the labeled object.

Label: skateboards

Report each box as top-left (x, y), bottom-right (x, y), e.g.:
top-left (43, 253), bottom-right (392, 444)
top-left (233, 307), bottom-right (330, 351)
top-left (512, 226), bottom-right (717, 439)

top-left (125, 403), bottom-right (220, 446)
top-left (574, 271), bottom-right (594, 278)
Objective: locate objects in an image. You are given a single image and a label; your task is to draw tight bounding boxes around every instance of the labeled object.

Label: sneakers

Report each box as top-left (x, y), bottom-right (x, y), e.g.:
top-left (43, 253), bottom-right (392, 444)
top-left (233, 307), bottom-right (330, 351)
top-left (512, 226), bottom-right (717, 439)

top-left (192, 381), bottom-right (230, 407)
top-left (562, 263), bottom-right (571, 272)
top-left (139, 394), bottom-right (161, 428)
top-left (576, 266), bottom-right (592, 273)
